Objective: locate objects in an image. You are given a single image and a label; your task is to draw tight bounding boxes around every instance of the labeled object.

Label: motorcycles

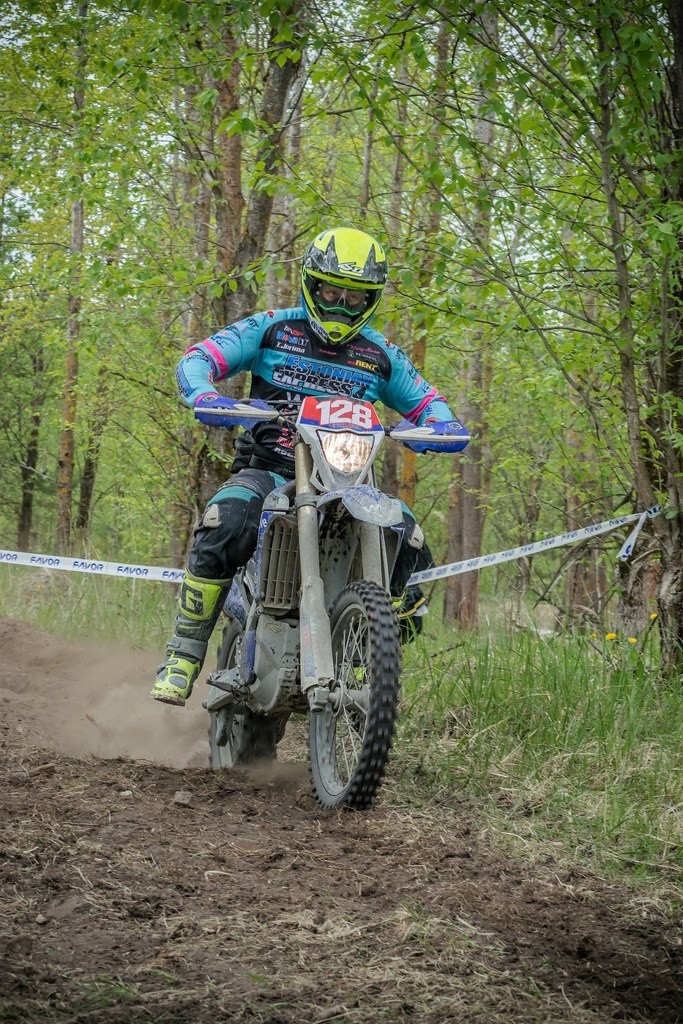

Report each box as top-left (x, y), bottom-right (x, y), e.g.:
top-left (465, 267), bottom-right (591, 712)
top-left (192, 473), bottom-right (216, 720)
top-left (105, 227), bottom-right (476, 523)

top-left (184, 393), bottom-right (472, 815)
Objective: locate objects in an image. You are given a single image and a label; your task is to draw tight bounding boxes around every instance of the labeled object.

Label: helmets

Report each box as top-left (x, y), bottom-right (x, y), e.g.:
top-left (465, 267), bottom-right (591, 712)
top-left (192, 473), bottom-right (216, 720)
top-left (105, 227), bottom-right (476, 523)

top-left (300, 227), bottom-right (390, 349)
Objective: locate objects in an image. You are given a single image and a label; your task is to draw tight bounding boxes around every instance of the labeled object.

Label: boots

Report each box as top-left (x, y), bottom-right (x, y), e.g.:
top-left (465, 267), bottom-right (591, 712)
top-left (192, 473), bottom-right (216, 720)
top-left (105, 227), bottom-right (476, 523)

top-left (150, 567), bottom-right (235, 706)
top-left (352, 592), bottom-right (408, 682)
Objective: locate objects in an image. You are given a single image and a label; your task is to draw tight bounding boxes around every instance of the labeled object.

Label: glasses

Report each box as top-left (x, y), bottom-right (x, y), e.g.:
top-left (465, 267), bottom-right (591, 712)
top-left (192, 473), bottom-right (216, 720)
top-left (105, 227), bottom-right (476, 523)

top-left (302, 262), bottom-right (387, 317)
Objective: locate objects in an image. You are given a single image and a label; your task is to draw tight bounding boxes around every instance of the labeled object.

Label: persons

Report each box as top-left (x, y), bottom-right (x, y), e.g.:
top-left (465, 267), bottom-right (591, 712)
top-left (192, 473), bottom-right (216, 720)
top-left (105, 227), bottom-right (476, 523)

top-left (149, 226), bottom-right (469, 706)
